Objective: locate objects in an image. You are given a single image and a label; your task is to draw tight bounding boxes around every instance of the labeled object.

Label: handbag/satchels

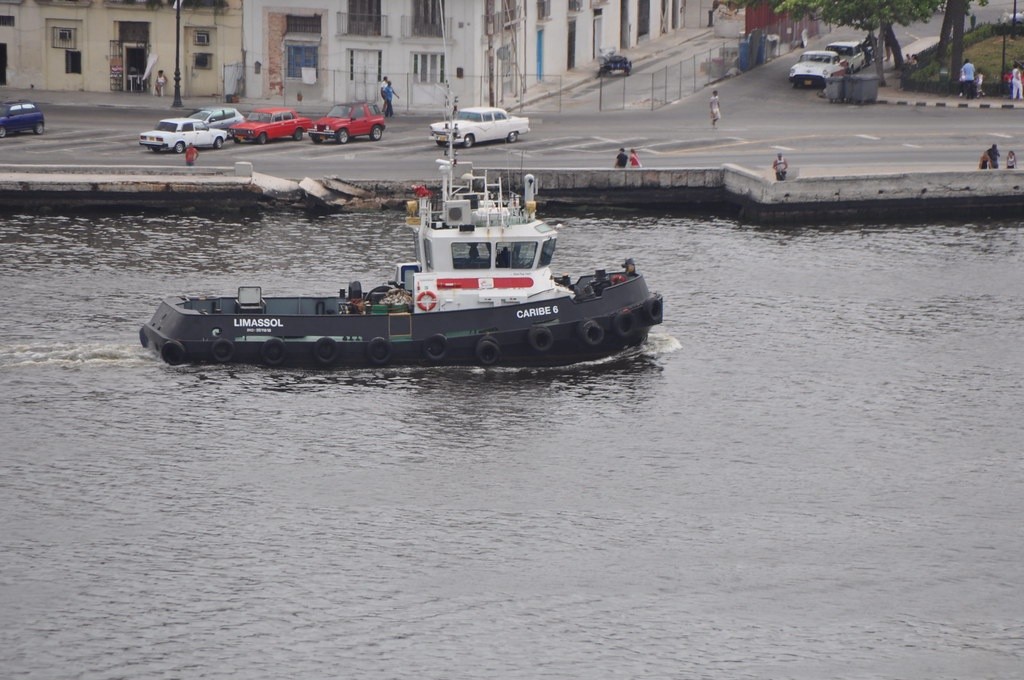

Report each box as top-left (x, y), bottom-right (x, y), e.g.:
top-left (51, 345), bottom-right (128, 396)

top-left (639, 164), bottom-right (642, 168)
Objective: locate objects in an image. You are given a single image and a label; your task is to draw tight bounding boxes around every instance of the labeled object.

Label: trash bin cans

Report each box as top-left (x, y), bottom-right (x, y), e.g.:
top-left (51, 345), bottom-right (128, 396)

top-left (843, 74), bottom-right (880, 104)
top-left (824, 77), bottom-right (843, 103)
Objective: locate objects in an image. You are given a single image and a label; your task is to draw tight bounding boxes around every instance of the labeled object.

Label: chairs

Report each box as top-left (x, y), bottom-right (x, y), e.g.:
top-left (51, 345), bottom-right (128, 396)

top-left (235, 286), bottom-right (267, 314)
top-left (153, 83), bottom-right (166, 96)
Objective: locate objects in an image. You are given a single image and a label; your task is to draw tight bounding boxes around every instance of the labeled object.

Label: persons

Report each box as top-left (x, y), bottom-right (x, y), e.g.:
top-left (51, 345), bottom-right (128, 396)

top-left (622, 259), bottom-right (639, 281)
top-left (615, 142), bottom-right (628, 170)
top-left (905, 54), bottom-right (918, 70)
top-left (1004, 59), bottom-right (1024, 99)
top-left (978, 144), bottom-right (1001, 170)
top-left (773, 152), bottom-right (788, 181)
top-left (959, 58), bottom-right (987, 99)
top-left (186, 143), bottom-right (198, 166)
top-left (381, 76), bottom-right (400, 117)
top-left (710, 90), bottom-right (721, 129)
top-left (882, 28), bottom-right (894, 63)
top-left (155, 69), bottom-right (168, 98)
top-left (1006, 151), bottom-right (1016, 169)
top-left (629, 148), bottom-right (641, 168)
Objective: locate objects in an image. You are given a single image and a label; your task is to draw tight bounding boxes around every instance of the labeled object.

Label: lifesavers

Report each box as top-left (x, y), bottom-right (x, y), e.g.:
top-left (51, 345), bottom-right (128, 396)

top-left (527, 324), bottom-right (554, 352)
top-left (260, 337), bottom-right (288, 366)
top-left (422, 334), bottom-right (450, 361)
top-left (417, 291), bottom-right (438, 312)
top-left (139, 328), bottom-right (148, 347)
top-left (642, 296), bottom-right (663, 324)
top-left (476, 335), bottom-right (500, 347)
top-left (312, 336), bottom-right (339, 365)
top-left (580, 319), bottom-right (606, 346)
top-left (210, 338), bottom-right (236, 363)
top-left (367, 337), bottom-right (395, 364)
top-left (161, 340), bottom-right (187, 365)
top-left (475, 340), bottom-right (501, 366)
top-left (612, 308), bottom-right (638, 339)
top-left (610, 275), bottom-right (627, 285)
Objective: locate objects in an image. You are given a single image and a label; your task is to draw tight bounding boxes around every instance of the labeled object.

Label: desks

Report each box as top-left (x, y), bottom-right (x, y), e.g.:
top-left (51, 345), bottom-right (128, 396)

top-left (128, 75), bottom-right (143, 92)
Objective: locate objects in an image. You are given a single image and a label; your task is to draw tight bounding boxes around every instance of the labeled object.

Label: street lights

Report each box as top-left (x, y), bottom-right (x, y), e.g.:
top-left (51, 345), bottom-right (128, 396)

top-left (1000, 12), bottom-right (1010, 96)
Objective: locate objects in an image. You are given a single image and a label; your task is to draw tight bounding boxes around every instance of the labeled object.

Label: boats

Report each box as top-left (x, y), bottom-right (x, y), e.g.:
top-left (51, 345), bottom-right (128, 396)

top-left (137, 119), bottom-right (663, 366)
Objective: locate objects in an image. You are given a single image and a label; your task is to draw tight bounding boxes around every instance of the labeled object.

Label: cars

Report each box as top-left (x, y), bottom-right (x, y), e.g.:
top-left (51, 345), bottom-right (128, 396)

top-left (428, 107), bottom-right (530, 148)
top-left (138, 118), bottom-right (227, 154)
top-left (188, 107), bottom-right (246, 140)
top-left (789, 50), bottom-right (848, 89)
top-left (825, 41), bottom-right (865, 74)
top-left (228, 108), bottom-right (315, 145)
top-left (0, 101), bottom-right (46, 138)
top-left (306, 102), bottom-right (386, 145)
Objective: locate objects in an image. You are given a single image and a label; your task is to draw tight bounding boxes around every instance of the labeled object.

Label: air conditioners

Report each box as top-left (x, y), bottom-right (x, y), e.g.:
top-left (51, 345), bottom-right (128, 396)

top-left (196, 34), bottom-right (208, 43)
top-left (444, 199), bottom-right (471, 226)
top-left (60, 31), bottom-right (71, 39)
